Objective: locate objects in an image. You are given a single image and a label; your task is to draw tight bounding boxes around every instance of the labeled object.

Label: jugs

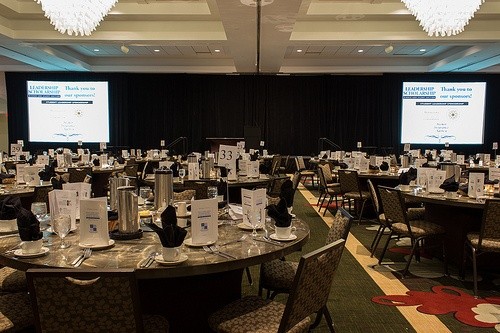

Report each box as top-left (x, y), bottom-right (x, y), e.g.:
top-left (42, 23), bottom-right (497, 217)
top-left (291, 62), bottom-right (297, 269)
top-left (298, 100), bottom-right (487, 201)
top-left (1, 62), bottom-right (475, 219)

top-left (117, 185), bottom-right (140, 234)
top-left (154, 166), bottom-right (173, 211)
top-left (109, 177), bottom-right (131, 212)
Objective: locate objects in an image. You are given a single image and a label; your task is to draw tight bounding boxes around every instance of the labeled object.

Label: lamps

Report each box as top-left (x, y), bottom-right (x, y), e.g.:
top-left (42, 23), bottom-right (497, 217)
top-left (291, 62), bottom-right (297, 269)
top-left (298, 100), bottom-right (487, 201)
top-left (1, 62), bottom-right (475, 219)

top-left (33, 0), bottom-right (119, 36)
top-left (400, 0), bottom-right (486, 37)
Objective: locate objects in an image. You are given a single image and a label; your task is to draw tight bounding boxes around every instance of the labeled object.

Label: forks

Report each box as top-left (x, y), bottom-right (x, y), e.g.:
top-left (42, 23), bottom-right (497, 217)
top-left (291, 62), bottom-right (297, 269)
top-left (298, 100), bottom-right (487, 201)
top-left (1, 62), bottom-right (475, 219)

top-left (74, 249), bottom-right (91, 268)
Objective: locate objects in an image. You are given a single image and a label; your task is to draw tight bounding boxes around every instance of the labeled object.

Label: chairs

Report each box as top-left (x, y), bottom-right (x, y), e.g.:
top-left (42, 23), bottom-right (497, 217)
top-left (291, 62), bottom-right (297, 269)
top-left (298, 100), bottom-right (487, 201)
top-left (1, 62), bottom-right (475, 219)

top-left (0, 150), bottom-right (353, 333)
top-left (293, 156), bottom-right (500, 296)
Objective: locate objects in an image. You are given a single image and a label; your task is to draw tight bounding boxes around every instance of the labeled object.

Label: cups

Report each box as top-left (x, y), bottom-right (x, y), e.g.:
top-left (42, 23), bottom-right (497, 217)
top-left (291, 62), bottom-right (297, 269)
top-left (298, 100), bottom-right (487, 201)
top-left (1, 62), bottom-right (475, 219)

top-left (380, 171), bottom-right (457, 198)
top-left (11, 153), bottom-right (298, 261)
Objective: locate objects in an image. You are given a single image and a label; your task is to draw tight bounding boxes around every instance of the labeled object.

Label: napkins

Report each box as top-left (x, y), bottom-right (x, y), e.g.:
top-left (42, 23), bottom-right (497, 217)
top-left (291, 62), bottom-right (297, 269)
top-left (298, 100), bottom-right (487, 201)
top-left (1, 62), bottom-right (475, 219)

top-left (407, 168), bottom-right (417, 180)
top-left (263, 204), bottom-right (293, 227)
top-left (439, 174), bottom-right (459, 191)
top-left (0, 195), bottom-right (22, 220)
top-left (17, 207), bottom-right (43, 241)
top-left (143, 204), bottom-right (187, 247)
top-left (399, 171), bottom-right (412, 184)
top-left (278, 180), bottom-right (295, 207)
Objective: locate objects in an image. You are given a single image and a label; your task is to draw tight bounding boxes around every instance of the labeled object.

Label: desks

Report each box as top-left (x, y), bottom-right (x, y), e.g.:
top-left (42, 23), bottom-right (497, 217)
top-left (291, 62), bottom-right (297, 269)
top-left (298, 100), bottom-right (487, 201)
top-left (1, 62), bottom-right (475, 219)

top-left (0, 180), bottom-right (53, 210)
top-left (332, 169), bottom-right (400, 219)
top-left (133, 157), bottom-right (162, 172)
top-left (143, 173), bottom-right (273, 204)
top-left (309, 158), bottom-right (335, 169)
top-left (43, 165), bottom-right (124, 196)
top-left (0, 203), bottom-right (311, 333)
top-left (402, 187), bottom-right (500, 271)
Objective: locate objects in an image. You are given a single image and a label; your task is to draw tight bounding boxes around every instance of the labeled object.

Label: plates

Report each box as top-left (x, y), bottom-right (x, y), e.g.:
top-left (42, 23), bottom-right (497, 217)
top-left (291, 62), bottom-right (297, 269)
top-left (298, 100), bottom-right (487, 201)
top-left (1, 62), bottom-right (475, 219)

top-left (238, 223), bottom-right (265, 230)
top-left (80, 239), bottom-right (114, 250)
top-left (154, 255), bottom-right (188, 266)
top-left (14, 247), bottom-right (49, 258)
top-left (176, 212), bottom-right (192, 217)
top-left (269, 235), bottom-right (297, 241)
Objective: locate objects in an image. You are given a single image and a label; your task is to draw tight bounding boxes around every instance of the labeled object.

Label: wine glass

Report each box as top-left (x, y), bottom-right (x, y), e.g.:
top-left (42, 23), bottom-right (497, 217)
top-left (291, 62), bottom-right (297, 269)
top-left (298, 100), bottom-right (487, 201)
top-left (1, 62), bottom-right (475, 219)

top-left (52, 216), bottom-right (71, 250)
top-left (31, 202), bottom-right (47, 232)
top-left (246, 207), bottom-right (261, 237)
top-left (139, 186), bottom-right (150, 209)
